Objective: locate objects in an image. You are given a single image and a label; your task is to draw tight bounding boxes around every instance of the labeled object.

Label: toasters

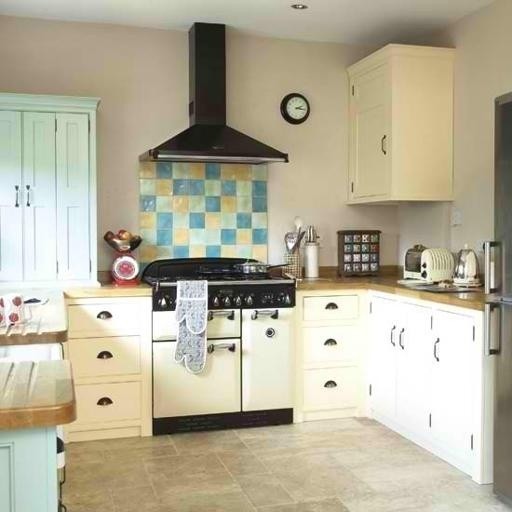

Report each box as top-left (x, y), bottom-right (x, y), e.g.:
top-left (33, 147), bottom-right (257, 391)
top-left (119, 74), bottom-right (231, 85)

top-left (402, 243), bottom-right (454, 284)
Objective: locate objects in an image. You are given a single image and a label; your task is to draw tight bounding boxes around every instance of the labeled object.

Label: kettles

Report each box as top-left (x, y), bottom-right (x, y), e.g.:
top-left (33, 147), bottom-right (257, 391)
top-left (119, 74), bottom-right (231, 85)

top-left (452, 242), bottom-right (481, 286)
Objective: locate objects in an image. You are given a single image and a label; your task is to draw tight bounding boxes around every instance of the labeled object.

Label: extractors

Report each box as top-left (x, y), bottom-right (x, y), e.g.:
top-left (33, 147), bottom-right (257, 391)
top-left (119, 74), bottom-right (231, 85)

top-left (138, 21), bottom-right (290, 165)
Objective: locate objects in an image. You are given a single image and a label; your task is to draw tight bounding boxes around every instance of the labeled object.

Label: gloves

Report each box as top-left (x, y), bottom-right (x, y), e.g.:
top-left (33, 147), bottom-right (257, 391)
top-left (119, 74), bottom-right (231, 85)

top-left (173, 280), bottom-right (207, 335)
top-left (174, 321), bottom-right (207, 373)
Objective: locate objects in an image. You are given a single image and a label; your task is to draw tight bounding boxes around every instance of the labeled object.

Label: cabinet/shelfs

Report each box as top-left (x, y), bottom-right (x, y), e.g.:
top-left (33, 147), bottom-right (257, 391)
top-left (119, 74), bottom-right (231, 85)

top-left (0, 361), bottom-right (78, 511)
top-left (360, 288), bottom-right (424, 447)
top-left (345, 38), bottom-right (456, 202)
top-left (420, 297), bottom-right (491, 484)
top-left (59, 288), bottom-right (154, 444)
top-left (0, 89), bottom-right (102, 285)
top-left (295, 285), bottom-right (365, 423)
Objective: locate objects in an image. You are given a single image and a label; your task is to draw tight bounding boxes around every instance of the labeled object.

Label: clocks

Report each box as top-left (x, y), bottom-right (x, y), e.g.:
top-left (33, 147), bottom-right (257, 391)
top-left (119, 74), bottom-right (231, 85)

top-left (280, 91), bottom-right (312, 125)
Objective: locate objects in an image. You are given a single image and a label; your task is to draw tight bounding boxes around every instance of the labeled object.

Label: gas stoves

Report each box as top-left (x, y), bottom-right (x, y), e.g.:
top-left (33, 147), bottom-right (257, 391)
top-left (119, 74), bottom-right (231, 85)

top-left (144, 273), bottom-right (294, 285)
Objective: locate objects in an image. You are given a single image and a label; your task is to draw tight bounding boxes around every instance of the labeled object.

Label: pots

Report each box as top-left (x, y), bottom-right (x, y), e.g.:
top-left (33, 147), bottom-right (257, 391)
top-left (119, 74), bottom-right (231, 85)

top-left (232, 259), bottom-right (291, 277)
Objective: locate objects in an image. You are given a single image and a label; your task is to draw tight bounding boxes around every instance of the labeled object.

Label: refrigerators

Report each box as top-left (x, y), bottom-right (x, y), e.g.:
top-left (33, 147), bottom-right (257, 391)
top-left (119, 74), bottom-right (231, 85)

top-left (483, 92), bottom-right (512, 511)
